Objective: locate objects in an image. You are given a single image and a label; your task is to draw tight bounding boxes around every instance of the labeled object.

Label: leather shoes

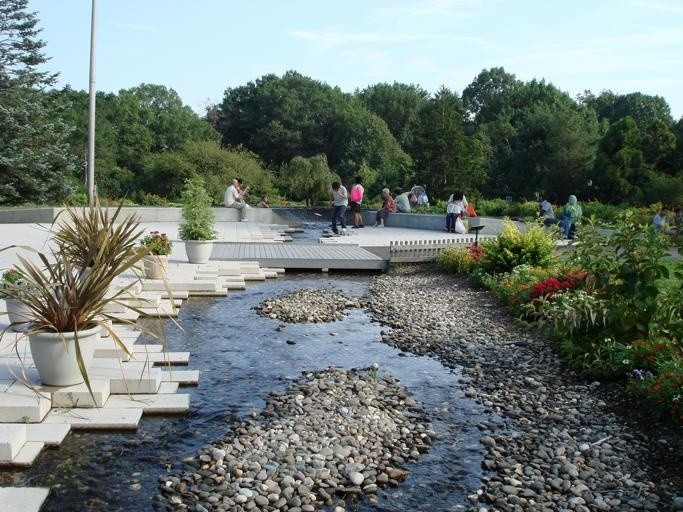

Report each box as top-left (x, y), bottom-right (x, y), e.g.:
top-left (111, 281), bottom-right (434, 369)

top-left (352, 224), bottom-right (364, 228)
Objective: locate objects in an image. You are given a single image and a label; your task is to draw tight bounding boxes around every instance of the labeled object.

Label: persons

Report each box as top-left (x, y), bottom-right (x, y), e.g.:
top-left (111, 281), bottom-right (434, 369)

top-left (348, 176), bottom-right (364, 228)
top-left (443, 190), bottom-right (468, 232)
top-left (653, 207), bottom-right (678, 247)
top-left (256, 196), bottom-right (269, 208)
top-left (373, 188), bottom-right (411, 228)
top-left (329, 182), bottom-right (348, 233)
top-left (539, 195), bottom-right (582, 239)
top-left (224, 178), bottom-right (250, 222)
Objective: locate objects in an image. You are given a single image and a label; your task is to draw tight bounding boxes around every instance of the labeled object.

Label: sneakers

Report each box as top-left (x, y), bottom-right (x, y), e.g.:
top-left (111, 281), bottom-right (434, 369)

top-left (331, 228), bottom-right (346, 236)
top-left (240, 218), bottom-right (248, 222)
top-left (444, 227), bottom-right (455, 232)
top-left (372, 223), bottom-right (384, 228)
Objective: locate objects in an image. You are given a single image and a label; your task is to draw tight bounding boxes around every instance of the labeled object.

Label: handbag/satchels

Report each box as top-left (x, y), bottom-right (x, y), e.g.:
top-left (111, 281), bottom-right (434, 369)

top-left (447, 203), bottom-right (460, 213)
top-left (351, 186), bottom-right (361, 202)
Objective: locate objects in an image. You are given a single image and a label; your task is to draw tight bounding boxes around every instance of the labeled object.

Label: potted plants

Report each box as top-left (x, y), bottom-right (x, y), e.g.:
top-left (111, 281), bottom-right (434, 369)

top-left (0, 188), bottom-right (186, 412)
top-left (177, 171), bottom-right (221, 264)
